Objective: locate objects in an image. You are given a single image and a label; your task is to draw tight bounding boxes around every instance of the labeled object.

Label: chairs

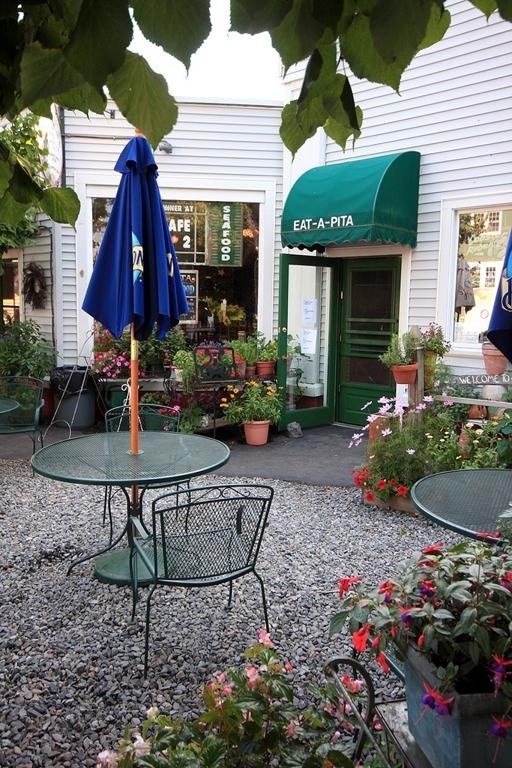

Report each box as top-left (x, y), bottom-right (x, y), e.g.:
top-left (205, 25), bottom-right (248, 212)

top-left (130, 484), bottom-right (270, 678)
top-left (0, 370), bottom-right (46, 477)
top-left (102, 403), bottom-right (191, 547)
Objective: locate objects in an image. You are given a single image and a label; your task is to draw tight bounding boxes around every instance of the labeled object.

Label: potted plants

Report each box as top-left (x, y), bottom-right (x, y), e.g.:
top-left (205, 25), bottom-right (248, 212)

top-left (377, 320), bottom-right (453, 390)
top-left (242, 330), bottom-right (276, 377)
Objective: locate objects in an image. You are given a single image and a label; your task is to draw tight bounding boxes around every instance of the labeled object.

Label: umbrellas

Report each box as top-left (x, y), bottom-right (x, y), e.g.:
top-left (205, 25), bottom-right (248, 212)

top-left (484, 229), bottom-right (511, 369)
top-left (76, 127), bottom-right (190, 452)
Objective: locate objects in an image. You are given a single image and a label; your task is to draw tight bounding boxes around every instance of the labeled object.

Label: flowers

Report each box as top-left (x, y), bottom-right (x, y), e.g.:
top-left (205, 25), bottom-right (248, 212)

top-left (91, 326), bottom-right (244, 377)
top-left (347, 394), bottom-right (512, 507)
top-left (219, 379), bottom-right (284, 424)
top-left (99, 629), bottom-right (359, 768)
top-left (331, 532), bottom-right (509, 740)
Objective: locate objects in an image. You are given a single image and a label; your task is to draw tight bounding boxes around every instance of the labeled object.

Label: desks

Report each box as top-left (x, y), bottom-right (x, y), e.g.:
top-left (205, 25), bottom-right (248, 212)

top-left (27, 428), bottom-right (236, 588)
top-left (411, 465), bottom-right (512, 550)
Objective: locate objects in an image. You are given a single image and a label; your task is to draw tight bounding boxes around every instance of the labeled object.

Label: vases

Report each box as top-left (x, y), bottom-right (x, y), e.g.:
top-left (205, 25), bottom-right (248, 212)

top-left (406, 644), bottom-right (510, 749)
top-left (244, 421), bottom-right (269, 446)
top-left (480, 343), bottom-right (509, 375)
